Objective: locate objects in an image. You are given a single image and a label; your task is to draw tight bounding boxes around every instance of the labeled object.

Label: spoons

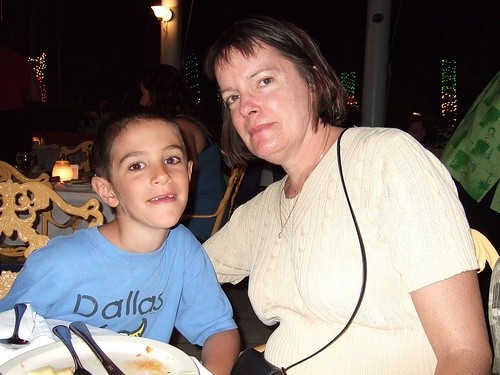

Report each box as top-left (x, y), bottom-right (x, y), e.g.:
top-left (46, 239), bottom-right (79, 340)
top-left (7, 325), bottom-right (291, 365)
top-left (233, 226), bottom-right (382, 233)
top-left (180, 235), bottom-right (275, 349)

top-left (0, 303), bottom-right (29, 349)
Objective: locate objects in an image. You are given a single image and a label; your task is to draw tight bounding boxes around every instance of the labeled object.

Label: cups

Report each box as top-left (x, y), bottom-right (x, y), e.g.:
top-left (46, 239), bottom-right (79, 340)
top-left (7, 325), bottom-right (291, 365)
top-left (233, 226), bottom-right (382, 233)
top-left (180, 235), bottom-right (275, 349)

top-left (71, 165), bottom-right (78, 179)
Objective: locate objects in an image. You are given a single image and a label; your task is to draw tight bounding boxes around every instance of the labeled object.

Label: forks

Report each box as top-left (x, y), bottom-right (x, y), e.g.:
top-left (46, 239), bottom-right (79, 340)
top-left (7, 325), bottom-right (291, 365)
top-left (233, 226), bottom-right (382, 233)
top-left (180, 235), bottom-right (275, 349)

top-left (52, 325), bottom-right (92, 375)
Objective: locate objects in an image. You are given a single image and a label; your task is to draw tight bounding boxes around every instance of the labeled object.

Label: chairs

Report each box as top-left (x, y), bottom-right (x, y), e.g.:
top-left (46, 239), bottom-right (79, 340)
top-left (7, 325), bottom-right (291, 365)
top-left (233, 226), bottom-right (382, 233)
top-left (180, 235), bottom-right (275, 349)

top-left (0, 140), bottom-right (245, 306)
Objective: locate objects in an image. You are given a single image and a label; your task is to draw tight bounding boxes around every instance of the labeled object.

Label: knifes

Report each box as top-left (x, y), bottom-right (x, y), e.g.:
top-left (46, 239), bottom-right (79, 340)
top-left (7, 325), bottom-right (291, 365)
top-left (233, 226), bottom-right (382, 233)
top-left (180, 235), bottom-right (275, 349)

top-left (69, 321), bottom-right (125, 375)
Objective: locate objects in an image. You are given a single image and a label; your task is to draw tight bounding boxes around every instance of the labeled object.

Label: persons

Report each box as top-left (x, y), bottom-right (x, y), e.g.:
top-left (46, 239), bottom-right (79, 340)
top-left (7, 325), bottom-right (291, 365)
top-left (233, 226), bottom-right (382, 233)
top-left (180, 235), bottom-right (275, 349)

top-left (140, 64), bottom-right (228, 242)
top-left (200, 15), bottom-right (493, 375)
top-left (404, 116), bottom-right (458, 156)
top-left (0, 108), bottom-right (241, 375)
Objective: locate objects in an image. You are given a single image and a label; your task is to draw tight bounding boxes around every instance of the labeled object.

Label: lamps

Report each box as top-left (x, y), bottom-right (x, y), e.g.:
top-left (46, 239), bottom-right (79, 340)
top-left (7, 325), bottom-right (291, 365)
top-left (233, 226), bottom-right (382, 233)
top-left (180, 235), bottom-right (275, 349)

top-left (151, 6), bottom-right (172, 31)
top-left (51, 154), bottom-right (74, 183)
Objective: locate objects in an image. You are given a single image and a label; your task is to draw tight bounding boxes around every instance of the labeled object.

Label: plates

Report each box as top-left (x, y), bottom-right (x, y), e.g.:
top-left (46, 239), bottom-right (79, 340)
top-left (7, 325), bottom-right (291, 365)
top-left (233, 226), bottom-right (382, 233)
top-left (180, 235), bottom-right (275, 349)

top-left (0, 336), bottom-right (200, 375)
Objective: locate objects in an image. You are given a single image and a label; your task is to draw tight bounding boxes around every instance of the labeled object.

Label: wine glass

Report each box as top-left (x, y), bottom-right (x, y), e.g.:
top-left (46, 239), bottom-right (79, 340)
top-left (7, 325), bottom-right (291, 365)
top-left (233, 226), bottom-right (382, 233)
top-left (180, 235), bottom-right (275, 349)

top-left (16, 152), bottom-right (36, 177)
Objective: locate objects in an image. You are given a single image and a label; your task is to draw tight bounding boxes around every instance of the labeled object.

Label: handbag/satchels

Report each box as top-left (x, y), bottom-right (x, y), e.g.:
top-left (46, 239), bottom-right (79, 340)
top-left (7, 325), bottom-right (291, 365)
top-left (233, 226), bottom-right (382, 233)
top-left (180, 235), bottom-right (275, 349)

top-left (231, 348), bottom-right (284, 375)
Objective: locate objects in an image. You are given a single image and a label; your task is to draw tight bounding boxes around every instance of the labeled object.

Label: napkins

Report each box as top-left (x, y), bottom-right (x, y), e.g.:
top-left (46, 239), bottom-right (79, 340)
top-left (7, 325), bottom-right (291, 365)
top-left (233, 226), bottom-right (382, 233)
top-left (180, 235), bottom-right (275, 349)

top-left (0, 304), bottom-right (56, 366)
